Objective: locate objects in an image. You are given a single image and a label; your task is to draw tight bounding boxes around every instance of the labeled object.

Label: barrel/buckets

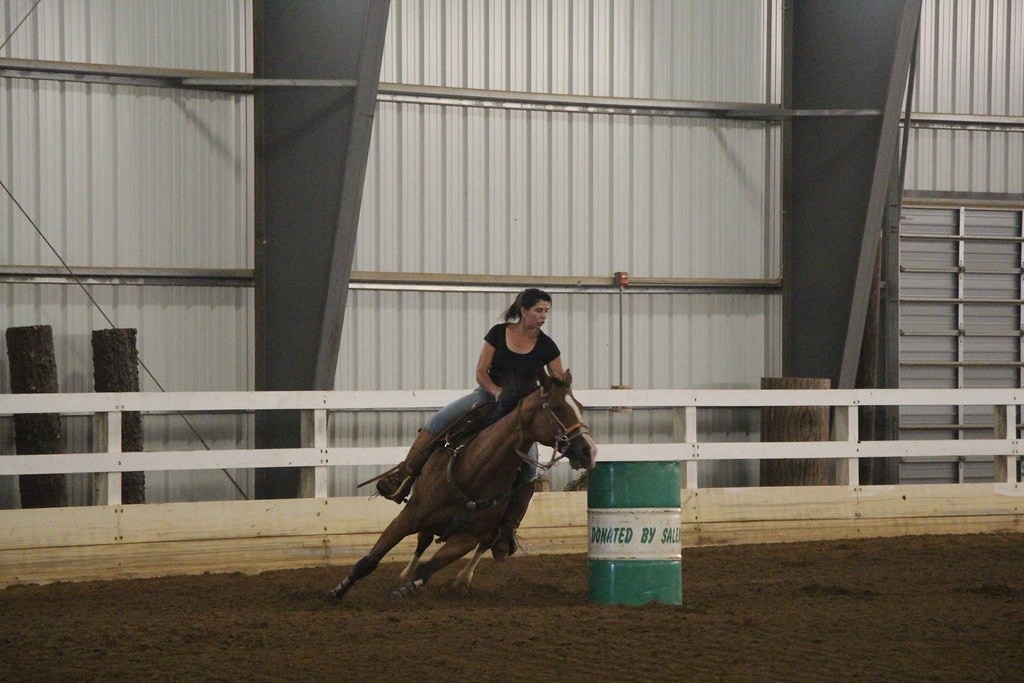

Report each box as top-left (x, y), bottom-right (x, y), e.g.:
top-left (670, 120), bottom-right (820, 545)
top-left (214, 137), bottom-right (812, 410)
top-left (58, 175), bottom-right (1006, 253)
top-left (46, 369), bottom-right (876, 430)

top-left (587, 454), bottom-right (684, 607)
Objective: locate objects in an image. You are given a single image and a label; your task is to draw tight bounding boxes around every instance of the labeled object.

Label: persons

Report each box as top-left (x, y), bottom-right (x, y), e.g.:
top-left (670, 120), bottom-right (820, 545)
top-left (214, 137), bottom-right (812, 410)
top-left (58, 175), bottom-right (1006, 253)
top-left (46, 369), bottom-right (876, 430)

top-left (376, 288), bottom-right (566, 560)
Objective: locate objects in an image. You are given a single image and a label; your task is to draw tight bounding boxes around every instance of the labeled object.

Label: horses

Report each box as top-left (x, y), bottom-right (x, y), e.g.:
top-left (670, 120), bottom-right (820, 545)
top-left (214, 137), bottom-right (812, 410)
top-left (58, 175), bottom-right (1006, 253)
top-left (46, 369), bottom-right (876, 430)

top-left (326, 368), bottom-right (598, 605)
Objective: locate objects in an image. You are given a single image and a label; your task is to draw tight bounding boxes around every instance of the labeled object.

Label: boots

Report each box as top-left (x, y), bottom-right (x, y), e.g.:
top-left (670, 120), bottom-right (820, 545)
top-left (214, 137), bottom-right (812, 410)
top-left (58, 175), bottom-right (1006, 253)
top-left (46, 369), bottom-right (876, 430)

top-left (490, 481), bottom-right (536, 564)
top-left (376, 428), bottom-right (439, 504)
